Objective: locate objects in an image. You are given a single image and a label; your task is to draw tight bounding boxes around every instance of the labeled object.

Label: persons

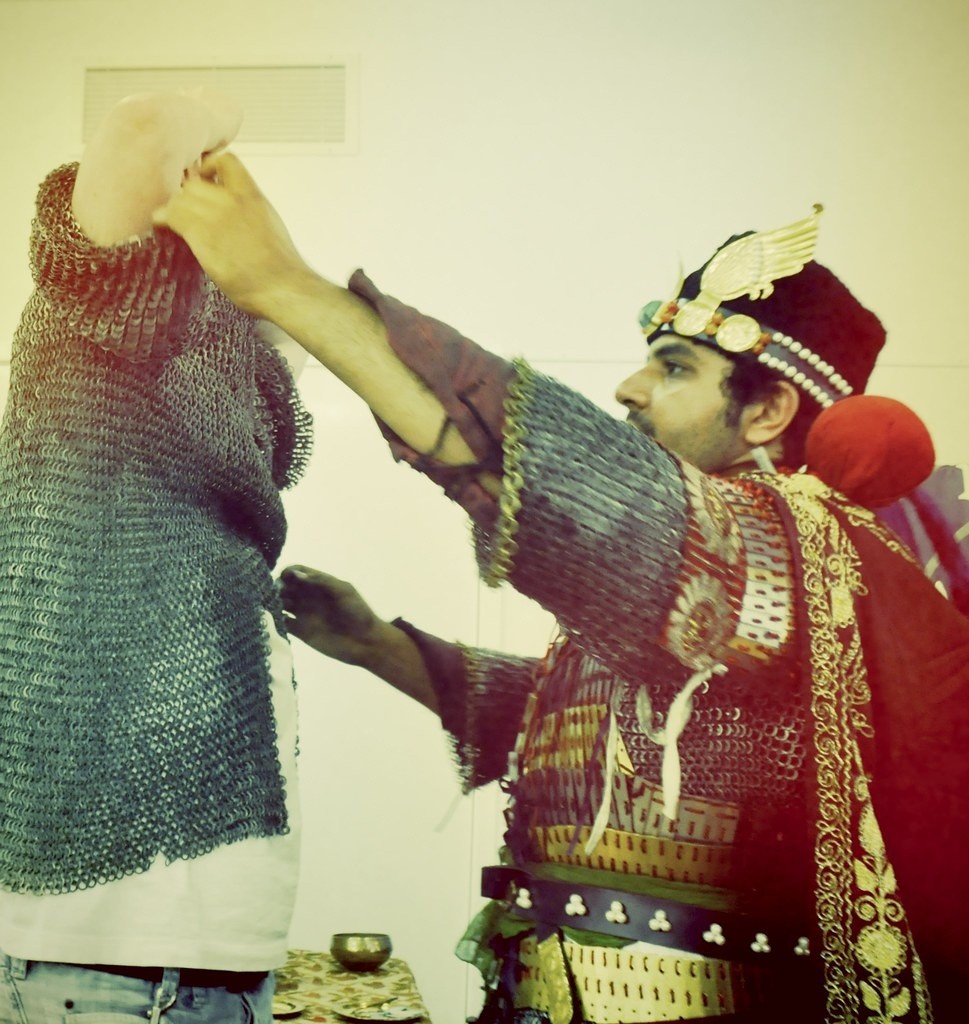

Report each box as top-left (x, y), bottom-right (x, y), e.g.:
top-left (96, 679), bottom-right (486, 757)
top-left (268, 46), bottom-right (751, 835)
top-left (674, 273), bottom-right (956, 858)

top-left (152, 153), bottom-right (969, 1024)
top-left (0, 93), bottom-right (318, 1024)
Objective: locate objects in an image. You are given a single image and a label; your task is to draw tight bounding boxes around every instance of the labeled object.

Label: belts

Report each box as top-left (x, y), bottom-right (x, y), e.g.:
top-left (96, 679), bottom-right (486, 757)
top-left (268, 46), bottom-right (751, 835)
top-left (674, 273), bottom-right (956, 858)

top-left (481, 865), bottom-right (809, 973)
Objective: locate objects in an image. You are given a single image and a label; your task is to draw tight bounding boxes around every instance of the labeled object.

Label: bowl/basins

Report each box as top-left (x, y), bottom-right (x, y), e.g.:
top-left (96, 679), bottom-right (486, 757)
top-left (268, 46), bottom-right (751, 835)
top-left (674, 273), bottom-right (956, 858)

top-left (330, 932), bottom-right (393, 972)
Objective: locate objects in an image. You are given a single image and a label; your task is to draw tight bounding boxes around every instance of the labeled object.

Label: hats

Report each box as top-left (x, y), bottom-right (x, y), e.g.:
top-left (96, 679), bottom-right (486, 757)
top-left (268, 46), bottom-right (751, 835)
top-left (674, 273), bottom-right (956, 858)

top-left (636, 201), bottom-right (887, 410)
